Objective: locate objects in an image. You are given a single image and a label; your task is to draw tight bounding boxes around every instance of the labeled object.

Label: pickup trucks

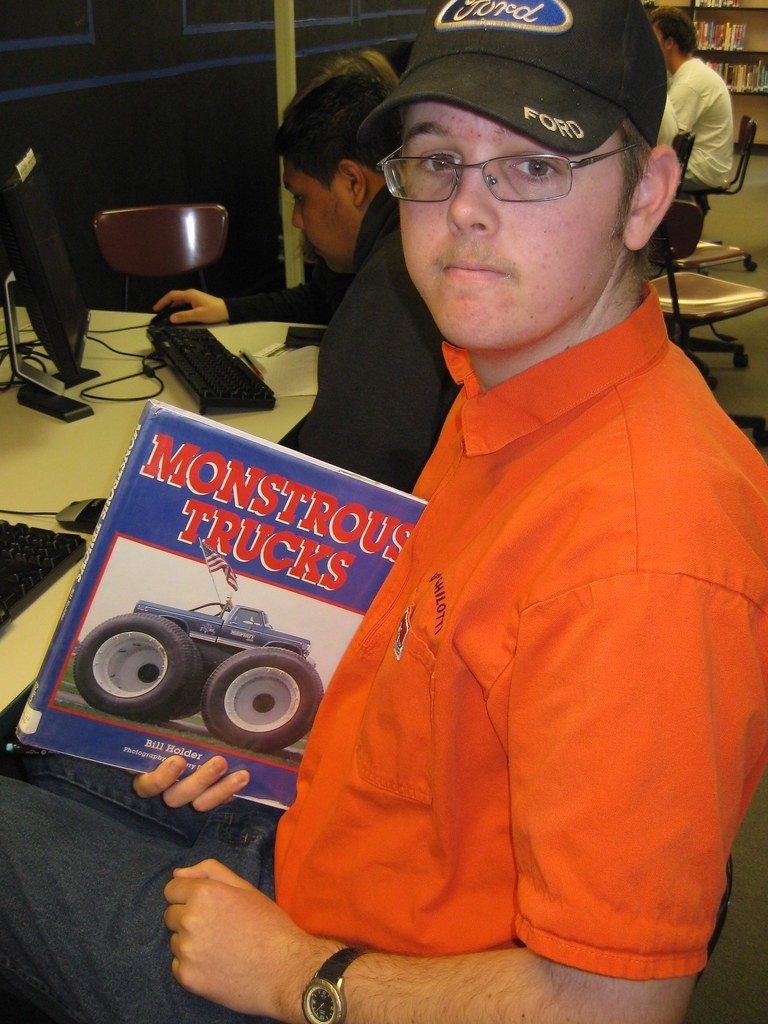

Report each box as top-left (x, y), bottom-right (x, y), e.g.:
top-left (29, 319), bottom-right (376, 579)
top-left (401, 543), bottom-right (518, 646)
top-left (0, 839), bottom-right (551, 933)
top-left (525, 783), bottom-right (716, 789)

top-left (73, 600), bottom-right (324, 750)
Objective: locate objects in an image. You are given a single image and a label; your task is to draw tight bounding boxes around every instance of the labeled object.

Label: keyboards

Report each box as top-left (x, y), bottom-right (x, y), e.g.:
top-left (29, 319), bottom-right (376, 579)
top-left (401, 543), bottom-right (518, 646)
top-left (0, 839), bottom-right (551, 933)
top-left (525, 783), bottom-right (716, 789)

top-left (147, 327), bottom-right (276, 416)
top-left (0, 520), bottom-right (86, 634)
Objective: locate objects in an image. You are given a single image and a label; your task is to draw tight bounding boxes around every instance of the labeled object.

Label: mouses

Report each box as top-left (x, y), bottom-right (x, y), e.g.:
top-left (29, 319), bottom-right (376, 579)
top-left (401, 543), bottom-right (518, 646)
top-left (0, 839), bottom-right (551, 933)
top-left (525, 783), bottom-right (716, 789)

top-left (150, 305), bottom-right (201, 328)
top-left (56, 498), bottom-right (107, 534)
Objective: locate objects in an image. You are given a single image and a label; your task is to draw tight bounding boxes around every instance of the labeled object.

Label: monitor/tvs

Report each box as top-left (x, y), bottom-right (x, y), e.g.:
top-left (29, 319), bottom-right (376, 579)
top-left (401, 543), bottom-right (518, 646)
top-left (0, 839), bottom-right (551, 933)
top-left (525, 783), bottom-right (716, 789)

top-left (0, 147), bottom-right (100, 423)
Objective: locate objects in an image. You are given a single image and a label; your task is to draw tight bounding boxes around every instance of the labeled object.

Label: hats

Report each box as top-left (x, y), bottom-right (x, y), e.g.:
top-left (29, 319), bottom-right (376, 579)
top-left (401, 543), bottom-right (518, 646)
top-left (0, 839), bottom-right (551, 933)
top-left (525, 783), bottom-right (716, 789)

top-left (356, 0), bottom-right (667, 152)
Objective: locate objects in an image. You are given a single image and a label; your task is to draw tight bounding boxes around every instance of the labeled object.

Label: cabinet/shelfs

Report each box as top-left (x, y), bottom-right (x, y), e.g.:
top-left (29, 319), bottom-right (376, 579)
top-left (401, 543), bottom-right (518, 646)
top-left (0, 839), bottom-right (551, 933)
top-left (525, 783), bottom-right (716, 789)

top-left (690, 0), bottom-right (768, 96)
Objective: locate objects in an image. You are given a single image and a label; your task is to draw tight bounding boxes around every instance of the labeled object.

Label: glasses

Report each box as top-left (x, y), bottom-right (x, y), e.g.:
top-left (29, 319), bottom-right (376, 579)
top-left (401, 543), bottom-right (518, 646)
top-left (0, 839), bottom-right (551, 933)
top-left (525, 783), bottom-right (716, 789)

top-left (377, 144), bottom-right (638, 203)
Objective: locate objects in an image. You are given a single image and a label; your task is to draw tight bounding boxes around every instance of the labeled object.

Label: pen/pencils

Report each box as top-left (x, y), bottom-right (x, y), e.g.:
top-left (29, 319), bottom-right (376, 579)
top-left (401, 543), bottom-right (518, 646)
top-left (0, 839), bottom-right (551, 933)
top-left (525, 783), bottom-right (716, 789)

top-left (239, 346), bottom-right (268, 381)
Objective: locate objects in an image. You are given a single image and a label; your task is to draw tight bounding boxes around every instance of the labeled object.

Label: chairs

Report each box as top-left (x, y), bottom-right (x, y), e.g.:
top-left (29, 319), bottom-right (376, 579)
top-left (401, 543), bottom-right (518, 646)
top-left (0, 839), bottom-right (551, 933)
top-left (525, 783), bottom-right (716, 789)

top-left (646, 130), bottom-right (696, 278)
top-left (652, 115), bottom-right (758, 277)
top-left (652, 200), bottom-right (768, 390)
top-left (93, 203), bottom-right (230, 313)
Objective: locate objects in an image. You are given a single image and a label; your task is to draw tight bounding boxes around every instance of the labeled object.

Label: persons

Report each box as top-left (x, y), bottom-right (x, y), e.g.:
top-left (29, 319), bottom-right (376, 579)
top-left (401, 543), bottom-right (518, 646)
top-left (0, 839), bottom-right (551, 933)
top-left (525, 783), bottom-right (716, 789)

top-left (0, 0), bottom-right (768, 1023)
top-left (649, 6), bottom-right (734, 191)
top-left (276, 52), bottom-right (460, 499)
top-left (153, 233), bottom-right (354, 323)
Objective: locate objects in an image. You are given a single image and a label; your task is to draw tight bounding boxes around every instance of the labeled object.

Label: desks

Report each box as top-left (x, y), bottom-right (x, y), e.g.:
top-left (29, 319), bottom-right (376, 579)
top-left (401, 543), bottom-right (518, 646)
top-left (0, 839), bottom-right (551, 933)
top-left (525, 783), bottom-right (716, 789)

top-left (0, 303), bottom-right (328, 736)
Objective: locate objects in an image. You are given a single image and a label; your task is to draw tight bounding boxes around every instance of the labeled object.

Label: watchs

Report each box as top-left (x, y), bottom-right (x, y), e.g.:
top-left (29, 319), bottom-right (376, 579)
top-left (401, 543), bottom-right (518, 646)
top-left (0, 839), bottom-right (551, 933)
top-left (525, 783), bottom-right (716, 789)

top-left (303, 946), bottom-right (362, 1024)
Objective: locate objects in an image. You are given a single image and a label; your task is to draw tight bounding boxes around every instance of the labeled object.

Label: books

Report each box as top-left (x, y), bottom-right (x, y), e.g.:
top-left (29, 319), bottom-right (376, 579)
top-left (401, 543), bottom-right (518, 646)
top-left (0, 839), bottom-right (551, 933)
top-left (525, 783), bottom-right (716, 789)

top-left (694, 21), bottom-right (746, 51)
top-left (12, 399), bottom-right (429, 810)
top-left (695, 0), bottom-right (739, 8)
top-left (706, 59), bottom-right (768, 93)
